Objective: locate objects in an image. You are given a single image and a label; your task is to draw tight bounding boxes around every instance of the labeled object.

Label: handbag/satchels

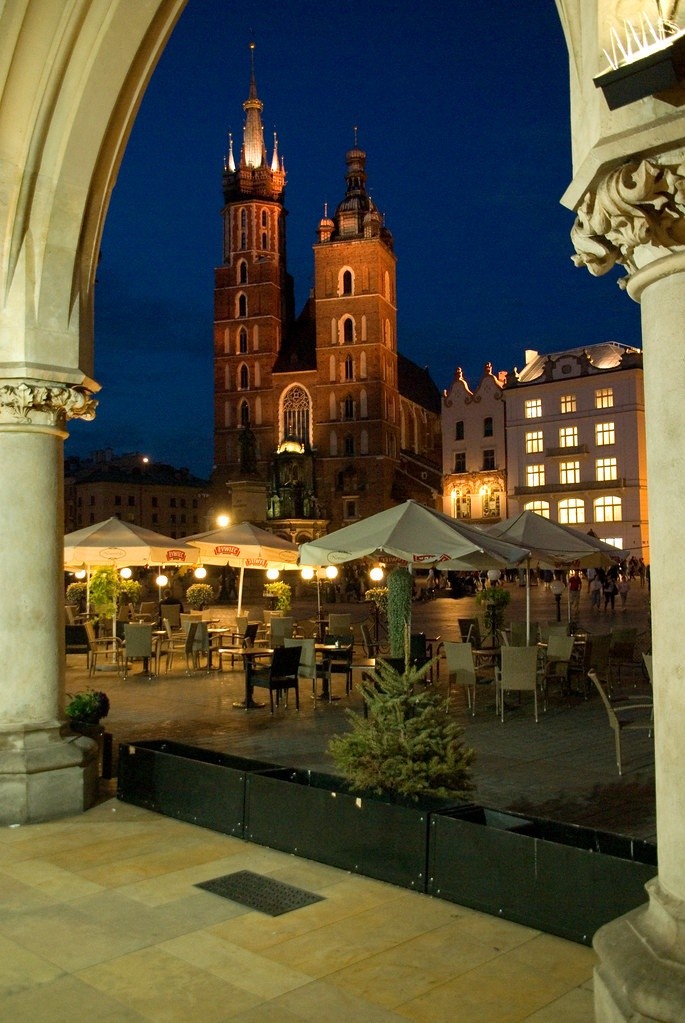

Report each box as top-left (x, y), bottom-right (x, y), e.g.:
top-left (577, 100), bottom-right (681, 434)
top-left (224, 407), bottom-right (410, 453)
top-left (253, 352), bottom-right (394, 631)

top-left (614, 586), bottom-right (618, 595)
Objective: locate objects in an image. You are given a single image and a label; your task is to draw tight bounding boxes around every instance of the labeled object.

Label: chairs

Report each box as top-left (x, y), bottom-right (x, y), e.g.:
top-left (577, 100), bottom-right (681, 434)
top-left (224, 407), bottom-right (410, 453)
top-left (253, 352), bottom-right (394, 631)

top-left (65, 604), bottom-right (654, 775)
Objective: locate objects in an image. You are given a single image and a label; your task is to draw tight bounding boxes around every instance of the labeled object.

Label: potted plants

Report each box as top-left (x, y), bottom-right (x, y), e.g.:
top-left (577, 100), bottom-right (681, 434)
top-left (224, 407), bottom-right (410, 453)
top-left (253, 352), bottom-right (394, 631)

top-left (262, 580), bottom-right (292, 624)
top-left (63, 687), bottom-right (110, 777)
top-left (186, 583), bottom-right (214, 620)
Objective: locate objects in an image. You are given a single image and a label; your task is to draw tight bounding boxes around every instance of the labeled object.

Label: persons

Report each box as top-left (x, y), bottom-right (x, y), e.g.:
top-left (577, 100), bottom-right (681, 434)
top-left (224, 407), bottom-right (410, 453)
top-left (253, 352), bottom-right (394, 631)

top-left (412, 562), bottom-right (650, 615)
top-left (566, 570), bottom-right (582, 615)
top-left (138, 566), bottom-right (196, 598)
top-left (155, 589), bottom-right (184, 630)
top-left (225, 568), bottom-right (239, 600)
top-left (335, 560), bottom-right (394, 604)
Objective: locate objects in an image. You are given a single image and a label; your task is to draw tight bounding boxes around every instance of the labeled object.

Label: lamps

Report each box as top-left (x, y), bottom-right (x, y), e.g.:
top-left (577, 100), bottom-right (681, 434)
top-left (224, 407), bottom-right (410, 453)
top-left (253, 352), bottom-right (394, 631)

top-left (487, 568), bottom-right (501, 669)
top-left (549, 579), bottom-right (565, 622)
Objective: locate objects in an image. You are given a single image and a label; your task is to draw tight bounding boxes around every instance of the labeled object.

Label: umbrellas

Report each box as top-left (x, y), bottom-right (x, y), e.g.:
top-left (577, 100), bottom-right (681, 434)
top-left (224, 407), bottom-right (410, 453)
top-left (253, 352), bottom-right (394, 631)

top-left (62, 516), bottom-right (199, 664)
top-left (175, 520), bottom-right (321, 645)
top-left (298, 497), bottom-right (532, 664)
top-left (481, 509), bottom-right (634, 633)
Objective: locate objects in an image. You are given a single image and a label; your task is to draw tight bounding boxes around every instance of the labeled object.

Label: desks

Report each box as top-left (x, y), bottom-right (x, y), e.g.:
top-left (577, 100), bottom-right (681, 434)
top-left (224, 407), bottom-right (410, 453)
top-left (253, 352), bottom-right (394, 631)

top-left (313, 644), bottom-right (350, 701)
top-left (217, 648), bottom-right (274, 710)
top-left (132, 630), bottom-right (167, 677)
top-left (536, 634), bottom-right (586, 691)
top-left (198, 629), bottom-right (231, 671)
top-left (471, 647), bottom-right (501, 708)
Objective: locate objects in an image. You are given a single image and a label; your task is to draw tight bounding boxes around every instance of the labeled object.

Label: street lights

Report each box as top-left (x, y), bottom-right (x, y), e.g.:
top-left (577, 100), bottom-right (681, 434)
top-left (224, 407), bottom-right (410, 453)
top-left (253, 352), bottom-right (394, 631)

top-left (549, 580), bottom-right (566, 623)
top-left (487, 569), bottom-right (502, 665)
top-left (369, 567), bottom-right (385, 653)
top-left (300, 566), bottom-right (339, 645)
top-left (266, 567), bottom-right (280, 611)
